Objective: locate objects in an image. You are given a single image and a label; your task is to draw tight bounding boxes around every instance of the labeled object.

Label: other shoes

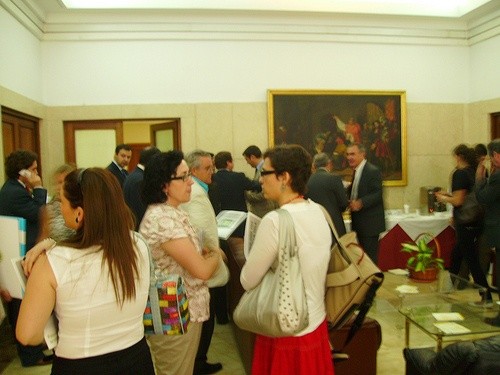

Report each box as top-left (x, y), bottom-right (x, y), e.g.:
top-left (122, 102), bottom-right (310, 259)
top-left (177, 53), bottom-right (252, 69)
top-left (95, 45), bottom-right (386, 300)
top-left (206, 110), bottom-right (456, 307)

top-left (35, 354), bottom-right (53, 366)
top-left (193, 363), bottom-right (221, 375)
top-left (484, 316), bottom-right (500, 325)
top-left (468, 301), bottom-right (494, 308)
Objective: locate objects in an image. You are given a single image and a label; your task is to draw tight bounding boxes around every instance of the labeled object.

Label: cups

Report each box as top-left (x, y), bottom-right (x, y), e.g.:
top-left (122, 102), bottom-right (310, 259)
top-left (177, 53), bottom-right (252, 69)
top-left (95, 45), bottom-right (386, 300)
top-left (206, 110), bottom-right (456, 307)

top-left (416, 208), bottom-right (420, 217)
top-left (403, 204), bottom-right (410, 213)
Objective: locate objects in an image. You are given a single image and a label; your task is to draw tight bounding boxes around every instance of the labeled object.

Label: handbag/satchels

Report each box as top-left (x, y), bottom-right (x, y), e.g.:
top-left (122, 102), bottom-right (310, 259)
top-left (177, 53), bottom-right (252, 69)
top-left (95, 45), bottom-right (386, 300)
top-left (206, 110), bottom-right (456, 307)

top-left (231, 207), bottom-right (310, 337)
top-left (132, 231), bottom-right (190, 336)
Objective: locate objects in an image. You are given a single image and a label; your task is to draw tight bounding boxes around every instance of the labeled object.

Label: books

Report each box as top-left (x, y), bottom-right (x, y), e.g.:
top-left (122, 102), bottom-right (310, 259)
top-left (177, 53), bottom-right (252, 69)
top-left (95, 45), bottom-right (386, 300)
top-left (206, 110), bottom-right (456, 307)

top-left (428, 188), bottom-right (447, 211)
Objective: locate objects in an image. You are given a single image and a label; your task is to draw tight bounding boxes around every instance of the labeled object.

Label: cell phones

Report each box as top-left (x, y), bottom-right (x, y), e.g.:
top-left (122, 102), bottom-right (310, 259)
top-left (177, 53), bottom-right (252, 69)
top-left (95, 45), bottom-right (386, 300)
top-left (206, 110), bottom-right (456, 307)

top-left (19, 169), bottom-right (31, 178)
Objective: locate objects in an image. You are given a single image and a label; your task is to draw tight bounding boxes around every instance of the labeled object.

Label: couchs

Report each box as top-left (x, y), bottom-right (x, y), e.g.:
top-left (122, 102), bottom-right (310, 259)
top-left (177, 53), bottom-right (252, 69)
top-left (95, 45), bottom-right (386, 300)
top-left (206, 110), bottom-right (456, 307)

top-left (403, 335), bottom-right (500, 375)
top-left (224, 236), bottom-right (382, 375)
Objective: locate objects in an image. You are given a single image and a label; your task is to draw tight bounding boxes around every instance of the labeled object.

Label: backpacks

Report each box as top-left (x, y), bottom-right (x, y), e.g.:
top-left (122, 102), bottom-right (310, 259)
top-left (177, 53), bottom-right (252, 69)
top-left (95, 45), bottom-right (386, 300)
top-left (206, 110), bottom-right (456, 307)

top-left (318, 203), bottom-right (384, 330)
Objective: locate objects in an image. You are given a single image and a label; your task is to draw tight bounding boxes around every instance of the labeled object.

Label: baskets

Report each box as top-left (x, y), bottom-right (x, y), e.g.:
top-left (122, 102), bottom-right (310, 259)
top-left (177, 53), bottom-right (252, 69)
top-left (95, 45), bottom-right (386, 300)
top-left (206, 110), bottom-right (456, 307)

top-left (405, 232), bottom-right (440, 280)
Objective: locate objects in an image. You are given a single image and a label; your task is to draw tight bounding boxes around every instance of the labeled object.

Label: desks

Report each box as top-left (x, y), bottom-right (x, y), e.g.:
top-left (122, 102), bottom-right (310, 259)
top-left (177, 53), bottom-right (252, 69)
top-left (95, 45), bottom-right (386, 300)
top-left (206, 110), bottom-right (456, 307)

top-left (343, 210), bottom-right (456, 272)
top-left (379, 268), bottom-right (500, 353)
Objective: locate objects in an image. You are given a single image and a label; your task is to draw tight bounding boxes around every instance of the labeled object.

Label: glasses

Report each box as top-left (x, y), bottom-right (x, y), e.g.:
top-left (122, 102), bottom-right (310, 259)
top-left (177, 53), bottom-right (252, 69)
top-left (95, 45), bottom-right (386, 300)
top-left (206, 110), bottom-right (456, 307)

top-left (260, 168), bottom-right (278, 177)
top-left (169, 172), bottom-right (191, 183)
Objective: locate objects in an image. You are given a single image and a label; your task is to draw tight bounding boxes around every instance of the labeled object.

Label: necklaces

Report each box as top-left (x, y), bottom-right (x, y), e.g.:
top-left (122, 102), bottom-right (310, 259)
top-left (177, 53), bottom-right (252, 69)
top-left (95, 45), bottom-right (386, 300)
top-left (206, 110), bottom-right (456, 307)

top-left (280, 195), bottom-right (304, 206)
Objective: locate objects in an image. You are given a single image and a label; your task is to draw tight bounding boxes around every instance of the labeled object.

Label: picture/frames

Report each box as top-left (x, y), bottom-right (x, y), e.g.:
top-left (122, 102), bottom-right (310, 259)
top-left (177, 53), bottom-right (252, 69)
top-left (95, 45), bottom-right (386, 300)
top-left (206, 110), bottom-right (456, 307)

top-left (267, 89), bottom-right (409, 187)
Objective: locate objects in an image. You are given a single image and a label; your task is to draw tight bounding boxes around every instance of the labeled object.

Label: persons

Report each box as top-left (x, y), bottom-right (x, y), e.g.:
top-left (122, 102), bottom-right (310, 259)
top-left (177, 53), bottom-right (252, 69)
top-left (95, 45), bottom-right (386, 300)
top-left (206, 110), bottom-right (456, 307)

top-left (16, 167), bottom-right (155, 375)
top-left (434, 139), bottom-right (500, 327)
top-left (346, 142), bottom-right (386, 264)
top-left (137, 151), bottom-right (225, 375)
top-left (240, 145), bottom-right (334, 375)
top-left (0, 144), bottom-right (349, 375)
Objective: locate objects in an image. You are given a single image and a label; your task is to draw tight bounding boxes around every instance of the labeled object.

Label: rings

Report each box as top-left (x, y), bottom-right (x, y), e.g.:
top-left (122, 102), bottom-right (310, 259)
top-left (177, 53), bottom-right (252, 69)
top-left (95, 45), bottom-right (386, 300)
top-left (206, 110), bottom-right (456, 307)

top-left (23, 257), bottom-right (26, 260)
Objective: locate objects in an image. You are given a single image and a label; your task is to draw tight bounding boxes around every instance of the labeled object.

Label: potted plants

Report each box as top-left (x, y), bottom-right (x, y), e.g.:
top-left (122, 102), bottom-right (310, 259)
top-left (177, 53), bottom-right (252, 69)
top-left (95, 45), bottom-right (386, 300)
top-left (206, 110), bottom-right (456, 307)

top-left (400, 231), bottom-right (445, 280)
